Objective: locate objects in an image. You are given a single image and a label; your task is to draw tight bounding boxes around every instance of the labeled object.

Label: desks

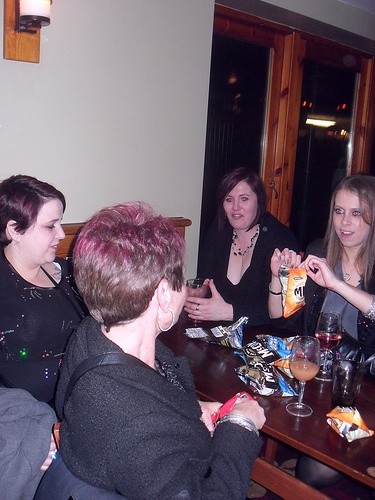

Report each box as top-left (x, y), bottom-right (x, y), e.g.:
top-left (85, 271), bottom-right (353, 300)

top-left (158, 318), bottom-right (375, 500)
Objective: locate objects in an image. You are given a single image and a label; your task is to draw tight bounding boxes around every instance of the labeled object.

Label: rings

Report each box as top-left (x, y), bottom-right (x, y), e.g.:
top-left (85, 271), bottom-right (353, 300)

top-left (196, 305), bottom-right (199, 310)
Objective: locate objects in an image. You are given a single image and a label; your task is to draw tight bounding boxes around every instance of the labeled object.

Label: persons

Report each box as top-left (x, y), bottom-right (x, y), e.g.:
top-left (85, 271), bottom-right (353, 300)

top-left (0, 174), bottom-right (91, 408)
top-left (182, 167), bottom-right (304, 333)
top-left (33, 203), bottom-right (267, 499)
top-left (266, 172), bottom-right (375, 490)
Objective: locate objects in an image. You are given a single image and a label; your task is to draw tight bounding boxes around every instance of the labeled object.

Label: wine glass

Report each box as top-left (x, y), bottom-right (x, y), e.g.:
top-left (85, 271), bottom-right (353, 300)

top-left (287, 337), bottom-right (320, 417)
top-left (314, 311), bottom-right (342, 382)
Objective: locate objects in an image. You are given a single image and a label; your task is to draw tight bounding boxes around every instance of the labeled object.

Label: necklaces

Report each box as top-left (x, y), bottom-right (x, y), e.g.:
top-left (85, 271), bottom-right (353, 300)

top-left (342, 259), bottom-right (352, 281)
top-left (231, 226), bottom-right (259, 256)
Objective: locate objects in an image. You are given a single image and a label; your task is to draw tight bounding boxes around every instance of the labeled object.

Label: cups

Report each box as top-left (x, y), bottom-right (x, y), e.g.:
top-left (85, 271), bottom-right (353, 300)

top-left (331, 359), bottom-right (364, 406)
top-left (186, 278), bottom-right (210, 324)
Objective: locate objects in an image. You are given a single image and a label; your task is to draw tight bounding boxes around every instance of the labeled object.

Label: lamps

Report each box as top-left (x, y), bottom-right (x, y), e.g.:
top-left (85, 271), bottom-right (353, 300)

top-left (15, 0), bottom-right (50, 33)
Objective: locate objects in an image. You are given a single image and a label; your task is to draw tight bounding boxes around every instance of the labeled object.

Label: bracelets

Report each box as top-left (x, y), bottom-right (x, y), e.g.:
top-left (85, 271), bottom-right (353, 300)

top-left (219, 412), bottom-right (260, 437)
top-left (361, 295), bottom-right (375, 322)
top-left (268, 282), bottom-right (282, 295)
